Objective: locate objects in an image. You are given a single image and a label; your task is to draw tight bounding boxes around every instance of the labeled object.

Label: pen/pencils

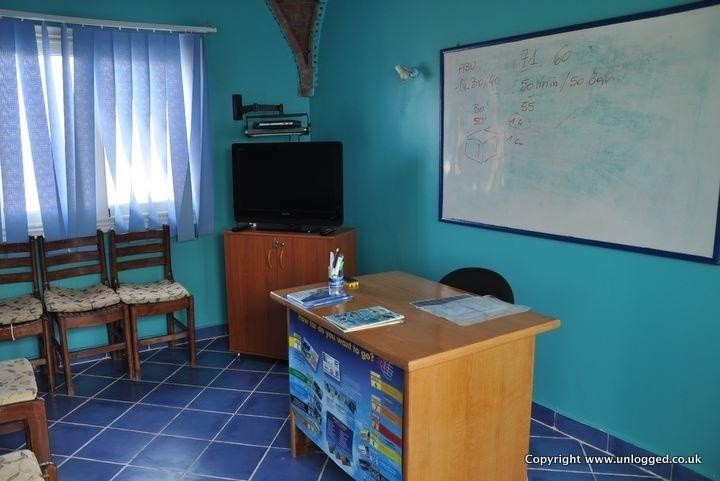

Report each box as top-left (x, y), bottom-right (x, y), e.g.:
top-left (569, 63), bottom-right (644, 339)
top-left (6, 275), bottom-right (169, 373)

top-left (329, 248), bottom-right (344, 274)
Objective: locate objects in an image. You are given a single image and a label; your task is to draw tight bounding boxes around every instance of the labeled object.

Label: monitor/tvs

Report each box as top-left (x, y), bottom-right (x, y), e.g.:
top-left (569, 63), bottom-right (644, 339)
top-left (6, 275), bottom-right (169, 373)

top-left (232, 141), bottom-right (343, 233)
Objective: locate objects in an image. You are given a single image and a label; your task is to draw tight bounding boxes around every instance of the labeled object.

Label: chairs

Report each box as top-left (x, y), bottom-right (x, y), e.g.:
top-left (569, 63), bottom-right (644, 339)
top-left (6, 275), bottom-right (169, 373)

top-left (438, 264), bottom-right (519, 306)
top-left (0, 223), bottom-right (201, 481)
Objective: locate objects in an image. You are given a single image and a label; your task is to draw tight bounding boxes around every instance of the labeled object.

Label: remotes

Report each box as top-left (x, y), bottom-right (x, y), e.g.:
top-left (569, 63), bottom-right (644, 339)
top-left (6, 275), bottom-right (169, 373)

top-left (320, 227), bottom-right (336, 234)
top-left (232, 224), bottom-right (249, 232)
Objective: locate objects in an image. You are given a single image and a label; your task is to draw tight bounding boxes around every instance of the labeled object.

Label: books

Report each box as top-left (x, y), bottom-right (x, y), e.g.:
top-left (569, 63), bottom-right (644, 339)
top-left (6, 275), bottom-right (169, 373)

top-left (324, 304), bottom-right (405, 332)
top-left (286, 285), bottom-right (355, 309)
top-left (325, 317), bottom-right (405, 334)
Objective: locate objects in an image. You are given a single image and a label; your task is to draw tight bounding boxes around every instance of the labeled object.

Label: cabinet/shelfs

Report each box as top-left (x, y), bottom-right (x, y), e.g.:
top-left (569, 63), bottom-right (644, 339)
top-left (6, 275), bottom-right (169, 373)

top-left (221, 226), bottom-right (356, 364)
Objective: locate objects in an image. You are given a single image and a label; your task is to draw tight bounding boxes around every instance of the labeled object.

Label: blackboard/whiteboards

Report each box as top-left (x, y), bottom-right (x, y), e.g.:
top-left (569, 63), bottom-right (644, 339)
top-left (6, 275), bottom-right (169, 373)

top-left (437, 1), bottom-right (720, 265)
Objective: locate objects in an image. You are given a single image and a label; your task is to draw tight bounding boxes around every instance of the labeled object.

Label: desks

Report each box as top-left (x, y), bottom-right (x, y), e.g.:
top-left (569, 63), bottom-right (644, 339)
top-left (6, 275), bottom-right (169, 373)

top-left (267, 268), bottom-right (562, 480)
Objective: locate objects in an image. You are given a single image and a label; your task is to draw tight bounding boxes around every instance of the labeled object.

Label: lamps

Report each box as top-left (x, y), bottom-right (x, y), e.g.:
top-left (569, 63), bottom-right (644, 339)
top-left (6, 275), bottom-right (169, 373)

top-left (394, 59), bottom-right (426, 84)
